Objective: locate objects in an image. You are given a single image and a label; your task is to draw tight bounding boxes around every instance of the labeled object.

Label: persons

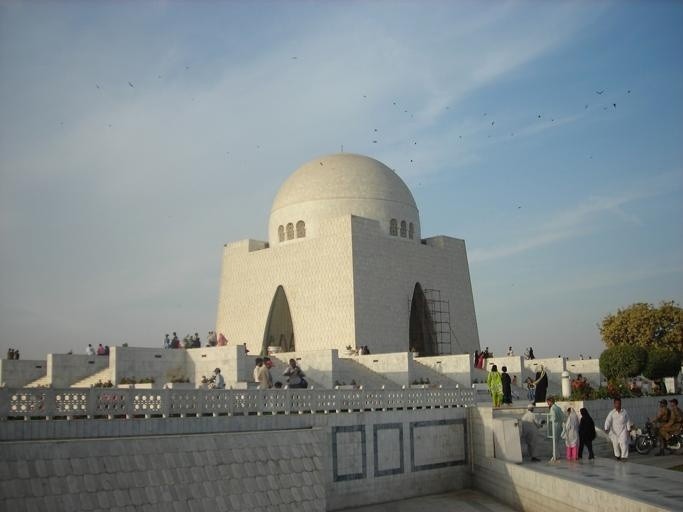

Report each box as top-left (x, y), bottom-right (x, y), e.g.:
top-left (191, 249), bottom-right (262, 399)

top-left (527, 378), bottom-right (535, 401)
top-left (547, 397), bottom-right (565, 425)
top-left (523, 348), bottom-right (529, 359)
top-left (560, 407), bottom-right (580, 460)
top-left (487, 364), bottom-right (505, 408)
top-left (213, 368), bottom-right (224, 389)
top-left (533, 365), bottom-right (549, 403)
top-left (363, 345), bottom-right (370, 355)
top-left (253, 357), bottom-right (308, 388)
top-left (6, 348), bottom-right (19, 360)
top-left (659, 399), bottom-right (683, 444)
top-left (164, 330), bottom-right (229, 349)
top-left (529, 347), bottom-right (535, 360)
top-left (645, 399), bottom-right (670, 456)
top-left (521, 403), bottom-right (543, 461)
top-left (506, 346), bottom-right (513, 356)
top-left (473, 347), bottom-right (489, 369)
top-left (604, 398), bottom-right (632, 462)
top-left (86, 343), bottom-right (109, 355)
top-left (578, 407), bottom-right (596, 460)
top-left (358, 346), bottom-right (363, 355)
top-left (500, 366), bottom-right (513, 407)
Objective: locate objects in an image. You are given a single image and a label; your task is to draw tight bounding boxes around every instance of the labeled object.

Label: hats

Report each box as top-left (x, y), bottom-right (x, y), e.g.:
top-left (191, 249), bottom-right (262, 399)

top-left (668, 399), bottom-right (679, 405)
top-left (658, 398), bottom-right (668, 405)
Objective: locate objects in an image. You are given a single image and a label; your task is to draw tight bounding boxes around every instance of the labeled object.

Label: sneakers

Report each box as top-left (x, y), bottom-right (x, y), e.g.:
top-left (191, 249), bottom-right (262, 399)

top-left (654, 449), bottom-right (666, 457)
top-left (574, 456), bottom-right (630, 463)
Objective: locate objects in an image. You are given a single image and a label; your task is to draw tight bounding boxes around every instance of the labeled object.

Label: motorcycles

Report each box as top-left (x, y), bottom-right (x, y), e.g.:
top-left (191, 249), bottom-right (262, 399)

top-left (635, 417), bottom-right (683, 454)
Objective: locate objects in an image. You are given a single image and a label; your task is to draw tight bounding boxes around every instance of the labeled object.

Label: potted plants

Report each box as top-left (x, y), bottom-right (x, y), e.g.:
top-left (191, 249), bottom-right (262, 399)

top-left (411, 347), bottom-right (419, 357)
top-left (342, 345), bottom-right (355, 356)
top-left (266, 336), bottom-right (282, 353)
top-left (116, 376), bottom-right (136, 390)
top-left (164, 376), bottom-right (195, 389)
top-left (134, 377), bottom-right (156, 389)
